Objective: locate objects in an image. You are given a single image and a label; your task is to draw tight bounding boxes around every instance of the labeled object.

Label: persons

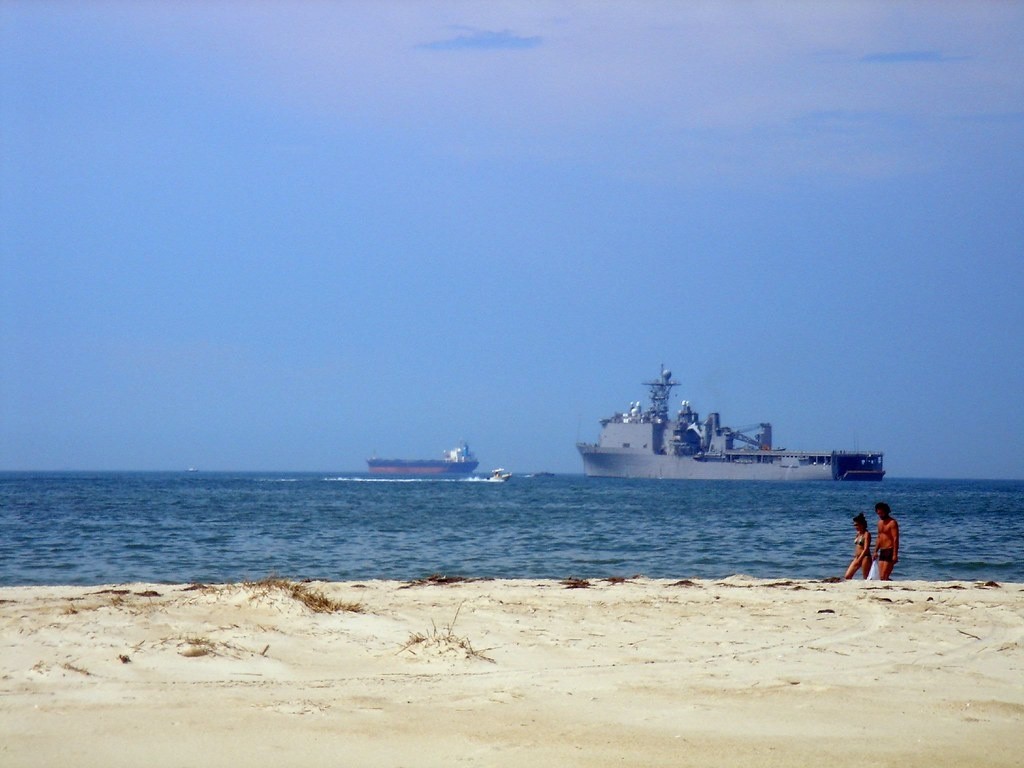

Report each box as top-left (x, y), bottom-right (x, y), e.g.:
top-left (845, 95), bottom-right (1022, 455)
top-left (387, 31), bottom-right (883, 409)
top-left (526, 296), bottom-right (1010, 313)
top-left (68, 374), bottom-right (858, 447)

top-left (844, 513), bottom-right (872, 579)
top-left (872, 502), bottom-right (899, 581)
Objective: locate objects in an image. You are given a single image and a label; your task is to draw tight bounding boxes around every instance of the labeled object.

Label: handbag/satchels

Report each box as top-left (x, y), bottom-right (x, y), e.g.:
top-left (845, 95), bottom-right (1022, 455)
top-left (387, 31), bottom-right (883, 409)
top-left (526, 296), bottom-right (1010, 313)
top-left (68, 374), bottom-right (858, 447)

top-left (866, 560), bottom-right (880, 580)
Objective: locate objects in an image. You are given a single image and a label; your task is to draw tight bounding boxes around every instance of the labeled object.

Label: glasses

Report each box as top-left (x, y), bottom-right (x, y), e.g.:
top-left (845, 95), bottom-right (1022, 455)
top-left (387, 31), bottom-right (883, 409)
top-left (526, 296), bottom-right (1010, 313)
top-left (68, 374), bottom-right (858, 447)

top-left (853, 524), bottom-right (860, 526)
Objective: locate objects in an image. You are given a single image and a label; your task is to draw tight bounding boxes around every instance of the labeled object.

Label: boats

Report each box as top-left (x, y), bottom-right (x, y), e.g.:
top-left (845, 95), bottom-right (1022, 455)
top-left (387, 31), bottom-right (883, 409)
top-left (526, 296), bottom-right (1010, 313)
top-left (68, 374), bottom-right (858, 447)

top-left (486, 467), bottom-right (513, 482)
top-left (571, 364), bottom-right (887, 486)
top-left (365, 441), bottom-right (479, 477)
